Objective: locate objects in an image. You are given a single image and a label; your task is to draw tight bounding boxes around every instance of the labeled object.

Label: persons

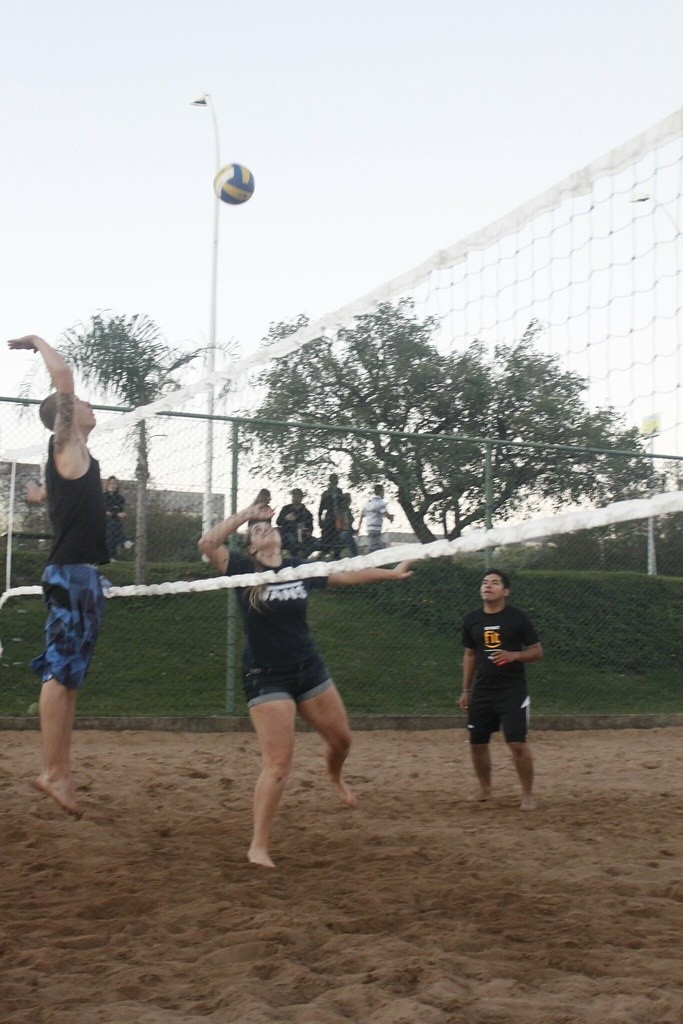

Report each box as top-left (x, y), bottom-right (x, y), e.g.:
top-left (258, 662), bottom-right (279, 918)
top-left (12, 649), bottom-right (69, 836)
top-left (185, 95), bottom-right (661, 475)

top-left (316, 473), bottom-right (358, 558)
top-left (275, 488), bottom-right (321, 559)
top-left (459, 569), bottom-right (543, 811)
top-left (358, 484), bottom-right (394, 553)
top-left (103, 476), bottom-right (135, 562)
top-left (7, 335), bottom-right (109, 815)
top-left (197, 502), bottom-right (414, 868)
top-left (247, 487), bottom-right (272, 523)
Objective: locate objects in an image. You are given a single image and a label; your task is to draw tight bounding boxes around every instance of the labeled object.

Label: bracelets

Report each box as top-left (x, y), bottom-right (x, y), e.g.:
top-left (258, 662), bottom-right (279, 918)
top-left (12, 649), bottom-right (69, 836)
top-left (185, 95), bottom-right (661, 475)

top-left (461, 688), bottom-right (470, 692)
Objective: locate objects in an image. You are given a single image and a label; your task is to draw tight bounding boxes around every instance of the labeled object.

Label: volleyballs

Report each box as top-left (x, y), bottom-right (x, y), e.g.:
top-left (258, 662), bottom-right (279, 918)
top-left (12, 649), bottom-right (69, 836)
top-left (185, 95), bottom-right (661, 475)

top-left (213, 163), bottom-right (255, 204)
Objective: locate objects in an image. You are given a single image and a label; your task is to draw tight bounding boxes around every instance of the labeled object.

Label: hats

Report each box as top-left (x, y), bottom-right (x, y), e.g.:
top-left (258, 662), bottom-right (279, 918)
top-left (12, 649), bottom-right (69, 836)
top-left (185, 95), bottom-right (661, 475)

top-left (290, 488), bottom-right (303, 496)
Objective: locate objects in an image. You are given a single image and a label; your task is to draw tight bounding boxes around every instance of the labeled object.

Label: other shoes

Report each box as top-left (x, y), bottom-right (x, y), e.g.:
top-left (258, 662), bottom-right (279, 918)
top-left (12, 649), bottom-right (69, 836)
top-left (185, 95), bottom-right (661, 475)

top-left (110, 558), bottom-right (117, 563)
top-left (124, 540), bottom-right (136, 549)
